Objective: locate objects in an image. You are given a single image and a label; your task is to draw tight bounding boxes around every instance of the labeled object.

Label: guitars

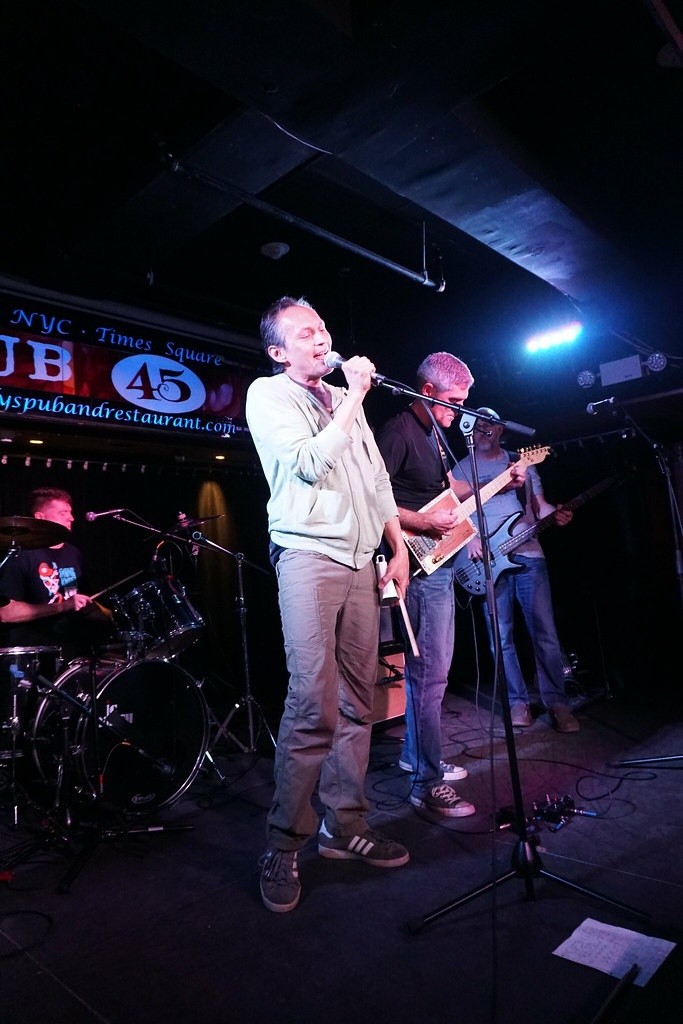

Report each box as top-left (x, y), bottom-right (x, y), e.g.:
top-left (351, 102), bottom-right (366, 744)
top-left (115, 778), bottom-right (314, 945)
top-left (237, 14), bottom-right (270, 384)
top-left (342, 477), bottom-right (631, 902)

top-left (401, 443), bottom-right (551, 576)
top-left (452, 459), bottom-right (640, 596)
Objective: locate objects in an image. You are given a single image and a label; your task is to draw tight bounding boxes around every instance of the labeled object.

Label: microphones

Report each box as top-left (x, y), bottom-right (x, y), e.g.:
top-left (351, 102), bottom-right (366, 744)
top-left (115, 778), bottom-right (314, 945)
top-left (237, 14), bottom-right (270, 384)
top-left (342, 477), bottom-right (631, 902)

top-left (379, 675), bottom-right (405, 684)
top-left (151, 548), bottom-right (159, 571)
top-left (153, 759), bottom-right (176, 780)
top-left (324, 351), bottom-right (387, 382)
top-left (86, 509), bottom-right (125, 522)
top-left (587, 397), bottom-right (618, 415)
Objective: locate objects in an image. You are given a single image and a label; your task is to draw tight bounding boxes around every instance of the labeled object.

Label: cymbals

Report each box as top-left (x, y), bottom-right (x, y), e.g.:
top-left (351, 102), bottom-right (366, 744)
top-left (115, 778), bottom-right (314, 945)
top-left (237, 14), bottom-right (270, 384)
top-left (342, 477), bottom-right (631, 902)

top-left (0, 517), bottom-right (73, 550)
top-left (142, 515), bottom-right (227, 544)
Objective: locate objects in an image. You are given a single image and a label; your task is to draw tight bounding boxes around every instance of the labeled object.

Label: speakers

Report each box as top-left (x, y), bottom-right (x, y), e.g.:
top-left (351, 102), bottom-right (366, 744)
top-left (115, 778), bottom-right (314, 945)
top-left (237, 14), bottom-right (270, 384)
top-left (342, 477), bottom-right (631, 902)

top-left (371, 640), bottom-right (408, 731)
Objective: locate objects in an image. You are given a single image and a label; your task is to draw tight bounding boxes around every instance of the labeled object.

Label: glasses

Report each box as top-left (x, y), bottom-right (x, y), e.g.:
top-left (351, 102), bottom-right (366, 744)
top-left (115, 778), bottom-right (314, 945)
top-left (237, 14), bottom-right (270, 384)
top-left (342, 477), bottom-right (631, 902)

top-left (473, 422), bottom-right (493, 431)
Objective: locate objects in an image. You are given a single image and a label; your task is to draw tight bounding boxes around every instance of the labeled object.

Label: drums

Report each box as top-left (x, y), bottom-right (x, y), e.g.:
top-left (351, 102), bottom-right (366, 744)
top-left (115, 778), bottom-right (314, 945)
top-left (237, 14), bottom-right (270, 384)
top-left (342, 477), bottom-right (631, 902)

top-left (108, 575), bottom-right (206, 661)
top-left (33, 653), bottom-right (210, 814)
top-left (0, 646), bottom-right (62, 759)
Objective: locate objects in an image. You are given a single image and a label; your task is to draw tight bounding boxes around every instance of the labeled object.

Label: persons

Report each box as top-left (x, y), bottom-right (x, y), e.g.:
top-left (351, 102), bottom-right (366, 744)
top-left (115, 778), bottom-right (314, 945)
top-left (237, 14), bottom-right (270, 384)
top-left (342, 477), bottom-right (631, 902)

top-left (452, 407), bottom-right (580, 733)
top-left (246, 298), bottom-right (409, 912)
top-left (0, 488), bottom-right (116, 646)
top-left (374, 352), bottom-right (525, 816)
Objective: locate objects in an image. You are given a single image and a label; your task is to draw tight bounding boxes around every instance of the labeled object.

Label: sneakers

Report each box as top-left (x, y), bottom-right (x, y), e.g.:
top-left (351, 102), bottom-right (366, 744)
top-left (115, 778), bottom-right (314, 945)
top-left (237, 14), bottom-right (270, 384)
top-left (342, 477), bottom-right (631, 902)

top-left (318, 818), bottom-right (409, 869)
top-left (410, 780), bottom-right (475, 818)
top-left (260, 847), bottom-right (301, 913)
top-left (509, 700), bottom-right (533, 726)
top-left (399, 749), bottom-right (468, 781)
top-left (550, 702), bottom-right (580, 733)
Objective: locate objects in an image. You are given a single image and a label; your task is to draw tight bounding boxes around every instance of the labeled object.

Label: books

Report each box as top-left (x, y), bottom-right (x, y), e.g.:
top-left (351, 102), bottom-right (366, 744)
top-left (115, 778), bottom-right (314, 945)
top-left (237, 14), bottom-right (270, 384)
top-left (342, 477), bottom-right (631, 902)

top-left (378, 579), bottom-right (420, 657)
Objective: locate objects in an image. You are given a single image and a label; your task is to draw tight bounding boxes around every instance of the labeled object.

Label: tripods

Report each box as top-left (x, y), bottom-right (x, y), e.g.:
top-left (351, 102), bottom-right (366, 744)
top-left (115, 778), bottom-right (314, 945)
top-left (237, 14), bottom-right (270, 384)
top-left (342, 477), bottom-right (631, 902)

top-left (190, 526), bottom-right (277, 753)
top-left (0, 674), bottom-right (196, 894)
top-left (370, 375), bottom-right (670, 940)
